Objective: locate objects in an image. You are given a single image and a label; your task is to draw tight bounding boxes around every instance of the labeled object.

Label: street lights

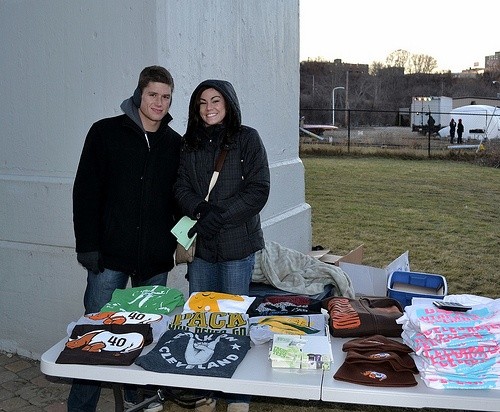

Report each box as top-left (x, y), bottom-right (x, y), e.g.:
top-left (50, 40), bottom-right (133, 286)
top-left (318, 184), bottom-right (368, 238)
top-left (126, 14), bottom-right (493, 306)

top-left (332, 86), bottom-right (345, 126)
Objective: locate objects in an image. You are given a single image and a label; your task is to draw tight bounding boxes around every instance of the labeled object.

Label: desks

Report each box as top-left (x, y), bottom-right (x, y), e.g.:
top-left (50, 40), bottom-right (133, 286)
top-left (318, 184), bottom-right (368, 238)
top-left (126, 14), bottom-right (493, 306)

top-left (38, 306), bottom-right (500, 412)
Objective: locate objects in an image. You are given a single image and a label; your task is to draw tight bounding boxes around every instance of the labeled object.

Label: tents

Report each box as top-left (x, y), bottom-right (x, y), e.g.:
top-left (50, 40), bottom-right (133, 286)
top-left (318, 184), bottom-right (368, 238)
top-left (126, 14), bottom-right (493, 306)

top-left (438, 105), bottom-right (500, 140)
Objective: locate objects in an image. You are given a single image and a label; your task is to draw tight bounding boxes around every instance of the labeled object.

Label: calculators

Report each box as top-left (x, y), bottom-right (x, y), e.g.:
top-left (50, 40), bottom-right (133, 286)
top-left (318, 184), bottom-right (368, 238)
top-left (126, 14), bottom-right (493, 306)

top-left (433, 300), bottom-right (472, 312)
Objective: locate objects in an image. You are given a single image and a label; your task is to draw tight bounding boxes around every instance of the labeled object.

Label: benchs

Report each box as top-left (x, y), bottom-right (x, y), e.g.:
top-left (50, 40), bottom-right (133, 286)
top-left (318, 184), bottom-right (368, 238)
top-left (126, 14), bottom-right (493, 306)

top-left (447, 144), bottom-right (479, 149)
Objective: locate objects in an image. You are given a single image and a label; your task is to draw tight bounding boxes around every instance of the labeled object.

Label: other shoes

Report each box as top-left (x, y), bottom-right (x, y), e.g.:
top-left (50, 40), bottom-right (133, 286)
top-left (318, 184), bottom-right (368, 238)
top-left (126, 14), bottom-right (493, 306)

top-left (124, 398), bottom-right (163, 412)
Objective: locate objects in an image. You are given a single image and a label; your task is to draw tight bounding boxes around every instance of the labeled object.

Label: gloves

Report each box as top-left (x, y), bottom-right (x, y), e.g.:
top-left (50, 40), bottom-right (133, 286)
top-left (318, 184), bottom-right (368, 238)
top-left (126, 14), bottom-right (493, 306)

top-left (188, 203), bottom-right (227, 250)
top-left (77, 251), bottom-right (105, 276)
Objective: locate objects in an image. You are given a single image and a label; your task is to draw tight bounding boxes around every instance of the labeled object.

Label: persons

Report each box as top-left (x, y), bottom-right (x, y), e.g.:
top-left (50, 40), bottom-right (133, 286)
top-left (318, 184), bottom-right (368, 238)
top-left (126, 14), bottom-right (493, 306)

top-left (173, 78), bottom-right (270, 299)
top-left (449, 118), bottom-right (456, 144)
top-left (66, 65), bottom-right (185, 412)
top-left (427, 115), bottom-right (436, 128)
top-left (457, 118), bottom-right (464, 145)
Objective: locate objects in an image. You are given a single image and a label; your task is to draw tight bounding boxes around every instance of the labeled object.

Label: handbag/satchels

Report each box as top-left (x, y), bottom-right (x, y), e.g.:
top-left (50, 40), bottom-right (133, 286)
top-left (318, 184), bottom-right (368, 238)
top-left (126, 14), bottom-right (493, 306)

top-left (176, 236), bottom-right (196, 264)
top-left (325, 295), bottom-right (404, 338)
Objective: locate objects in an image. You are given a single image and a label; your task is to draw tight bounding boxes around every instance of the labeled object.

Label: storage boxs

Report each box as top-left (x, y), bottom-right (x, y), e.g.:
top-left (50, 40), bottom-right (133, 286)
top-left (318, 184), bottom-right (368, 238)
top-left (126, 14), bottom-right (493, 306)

top-left (387, 270), bottom-right (448, 313)
top-left (270, 313), bottom-right (334, 371)
top-left (307, 244), bottom-right (364, 268)
top-left (336, 243), bottom-right (409, 298)
